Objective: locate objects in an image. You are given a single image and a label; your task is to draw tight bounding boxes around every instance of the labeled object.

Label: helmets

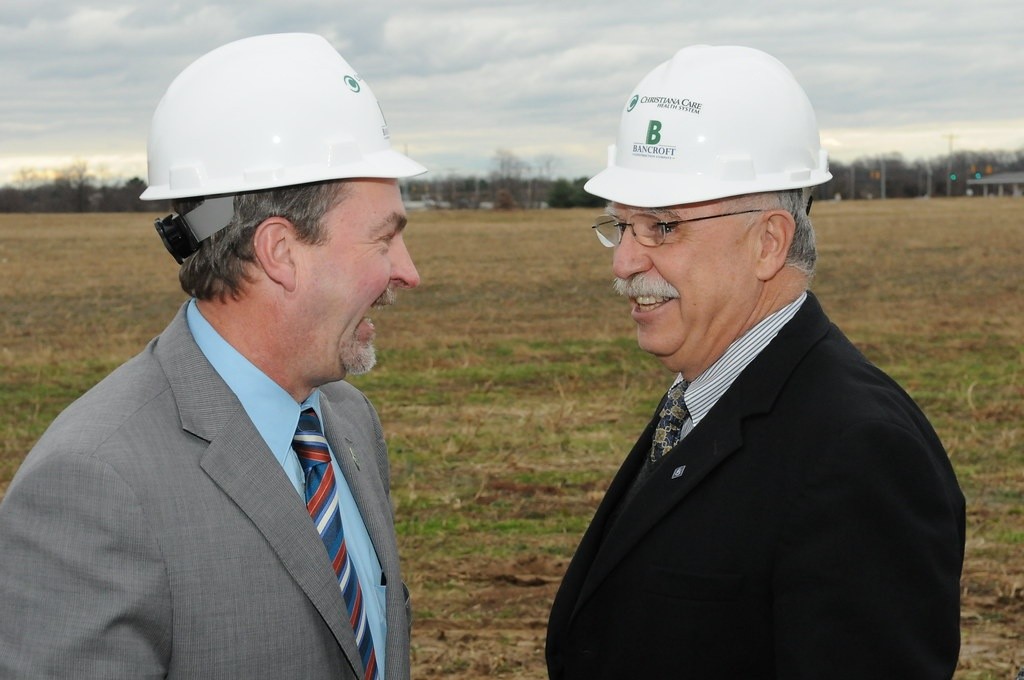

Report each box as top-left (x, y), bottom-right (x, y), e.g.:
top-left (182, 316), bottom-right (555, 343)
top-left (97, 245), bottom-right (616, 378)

top-left (138, 32), bottom-right (427, 200)
top-left (583, 44), bottom-right (833, 208)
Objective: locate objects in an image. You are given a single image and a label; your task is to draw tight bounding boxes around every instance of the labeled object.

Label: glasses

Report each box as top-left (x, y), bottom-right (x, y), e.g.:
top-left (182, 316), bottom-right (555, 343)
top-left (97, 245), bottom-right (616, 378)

top-left (591, 208), bottom-right (775, 248)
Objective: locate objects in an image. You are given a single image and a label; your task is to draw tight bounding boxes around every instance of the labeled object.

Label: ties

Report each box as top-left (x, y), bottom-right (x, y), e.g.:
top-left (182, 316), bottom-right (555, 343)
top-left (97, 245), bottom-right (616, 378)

top-left (291, 413), bottom-right (379, 680)
top-left (650, 378), bottom-right (689, 464)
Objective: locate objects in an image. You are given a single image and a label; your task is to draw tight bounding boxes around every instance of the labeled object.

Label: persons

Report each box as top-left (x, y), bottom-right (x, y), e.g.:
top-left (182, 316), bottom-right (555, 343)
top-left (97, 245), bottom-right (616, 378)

top-left (545, 44), bottom-right (966, 680)
top-left (0, 32), bottom-right (426, 680)
